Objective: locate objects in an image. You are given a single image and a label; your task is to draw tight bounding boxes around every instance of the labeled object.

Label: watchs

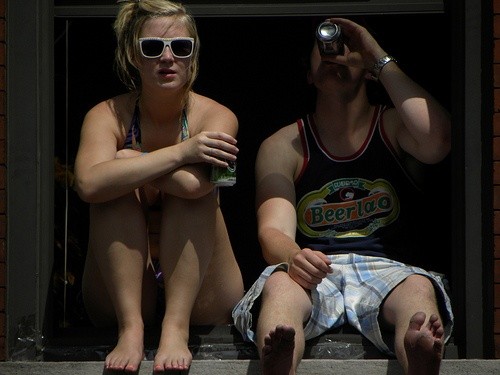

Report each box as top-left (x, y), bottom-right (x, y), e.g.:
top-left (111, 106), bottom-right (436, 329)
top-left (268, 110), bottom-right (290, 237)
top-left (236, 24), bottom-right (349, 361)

top-left (373, 56), bottom-right (397, 77)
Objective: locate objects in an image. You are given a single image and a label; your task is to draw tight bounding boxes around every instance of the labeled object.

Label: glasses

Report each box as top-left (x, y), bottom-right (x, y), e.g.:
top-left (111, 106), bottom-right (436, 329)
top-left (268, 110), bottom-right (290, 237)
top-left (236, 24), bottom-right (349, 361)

top-left (139, 38), bottom-right (194, 58)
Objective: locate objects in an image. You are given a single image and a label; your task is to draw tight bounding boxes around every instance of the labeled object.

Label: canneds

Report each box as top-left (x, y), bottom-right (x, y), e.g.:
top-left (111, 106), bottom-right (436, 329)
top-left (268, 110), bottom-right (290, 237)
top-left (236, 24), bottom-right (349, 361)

top-left (209, 151), bottom-right (236, 187)
top-left (317, 22), bottom-right (345, 56)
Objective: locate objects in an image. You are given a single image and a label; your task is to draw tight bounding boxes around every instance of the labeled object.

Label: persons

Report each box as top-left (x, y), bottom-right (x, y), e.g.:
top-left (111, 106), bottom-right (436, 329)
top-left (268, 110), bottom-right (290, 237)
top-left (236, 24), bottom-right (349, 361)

top-left (74, 0), bottom-right (245, 370)
top-left (255, 18), bottom-right (454, 375)
